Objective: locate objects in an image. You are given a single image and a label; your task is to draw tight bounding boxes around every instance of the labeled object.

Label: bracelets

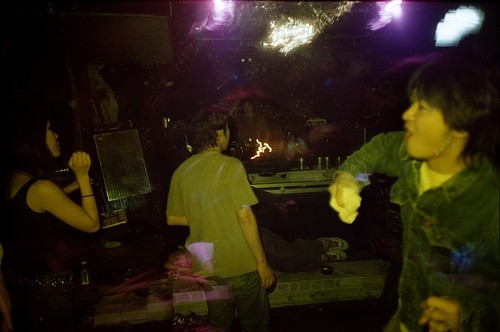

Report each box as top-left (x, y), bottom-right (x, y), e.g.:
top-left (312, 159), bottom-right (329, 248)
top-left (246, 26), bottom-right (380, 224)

top-left (81, 194), bottom-right (95, 197)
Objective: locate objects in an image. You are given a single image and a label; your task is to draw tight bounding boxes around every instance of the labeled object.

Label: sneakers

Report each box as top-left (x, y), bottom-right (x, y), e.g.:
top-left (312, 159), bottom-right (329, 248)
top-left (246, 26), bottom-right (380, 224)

top-left (321, 250), bottom-right (347, 262)
top-left (319, 237), bottom-right (348, 252)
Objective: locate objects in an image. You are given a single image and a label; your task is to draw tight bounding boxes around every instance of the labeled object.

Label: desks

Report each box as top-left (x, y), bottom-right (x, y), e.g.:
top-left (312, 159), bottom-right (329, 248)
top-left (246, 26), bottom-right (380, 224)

top-left (247, 179), bottom-right (390, 242)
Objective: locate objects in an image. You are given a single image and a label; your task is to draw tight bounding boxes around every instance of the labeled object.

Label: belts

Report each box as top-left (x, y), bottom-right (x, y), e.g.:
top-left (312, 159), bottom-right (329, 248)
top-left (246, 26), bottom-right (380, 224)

top-left (23, 275), bottom-right (71, 288)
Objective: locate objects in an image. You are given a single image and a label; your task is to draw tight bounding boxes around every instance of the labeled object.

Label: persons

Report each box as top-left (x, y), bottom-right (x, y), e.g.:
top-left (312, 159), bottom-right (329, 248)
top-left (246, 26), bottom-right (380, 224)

top-left (165, 54), bottom-right (500, 332)
top-left (0, 100), bottom-right (100, 332)
